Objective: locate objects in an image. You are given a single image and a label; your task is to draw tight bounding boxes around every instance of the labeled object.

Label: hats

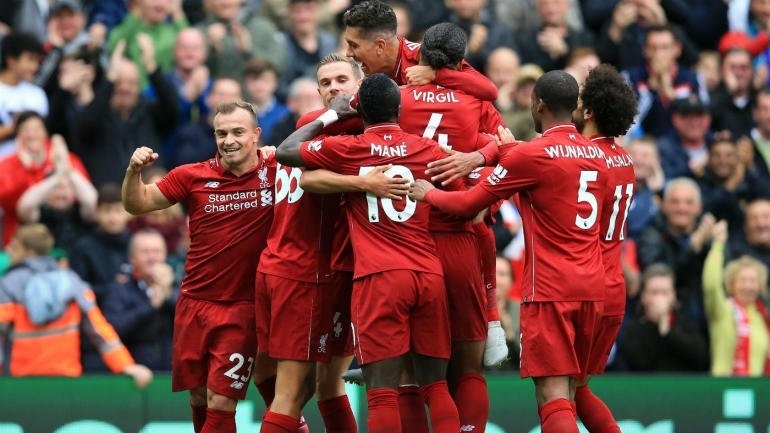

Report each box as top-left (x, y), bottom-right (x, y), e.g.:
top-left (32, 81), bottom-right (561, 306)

top-left (669, 93), bottom-right (714, 112)
top-left (512, 62), bottom-right (545, 91)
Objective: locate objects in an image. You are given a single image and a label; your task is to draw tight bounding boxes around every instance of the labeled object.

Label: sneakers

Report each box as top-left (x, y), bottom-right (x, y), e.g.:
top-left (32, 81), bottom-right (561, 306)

top-left (341, 367), bottom-right (367, 387)
top-left (482, 320), bottom-right (509, 371)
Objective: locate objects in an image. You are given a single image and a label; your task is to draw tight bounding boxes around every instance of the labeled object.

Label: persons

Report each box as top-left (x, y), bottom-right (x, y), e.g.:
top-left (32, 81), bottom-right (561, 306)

top-left (477, 63), bottom-right (644, 433)
top-left (339, 1), bottom-right (503, 104)
top-left (123, 102), bottom-right (278, 433)
top-left (279, 75), bottom-right (483, 433)
top-left (393, 21), bottom-right (513, 433)
top-left (409, 70), bottom-right (626, 433)
top-left (314, 53), bottom-right (363, 110)
top-left (0, 0), bottom-right (769, 390)
top-left (253, 158), bottom-right (411, 433)
